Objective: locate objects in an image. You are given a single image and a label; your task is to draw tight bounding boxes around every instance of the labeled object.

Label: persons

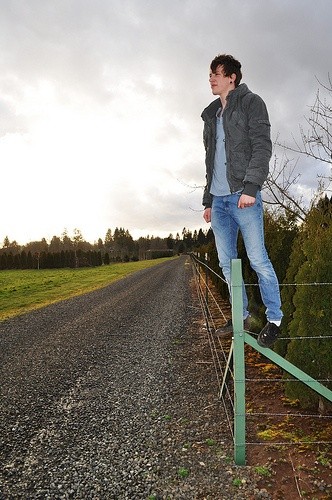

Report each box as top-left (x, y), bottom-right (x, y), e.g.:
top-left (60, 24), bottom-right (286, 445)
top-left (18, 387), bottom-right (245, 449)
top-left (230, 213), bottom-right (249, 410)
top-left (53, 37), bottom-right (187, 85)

top-left (201, 55), bottom-right (283, 348)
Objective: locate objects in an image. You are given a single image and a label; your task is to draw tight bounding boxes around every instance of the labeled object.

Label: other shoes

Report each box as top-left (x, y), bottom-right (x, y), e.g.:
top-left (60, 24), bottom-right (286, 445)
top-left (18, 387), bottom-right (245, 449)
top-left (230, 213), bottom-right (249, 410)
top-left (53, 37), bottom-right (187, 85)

top-left (257, 322), bottom-right (279, 348)
top-left (215, 318), bottom-right (250, 338)
